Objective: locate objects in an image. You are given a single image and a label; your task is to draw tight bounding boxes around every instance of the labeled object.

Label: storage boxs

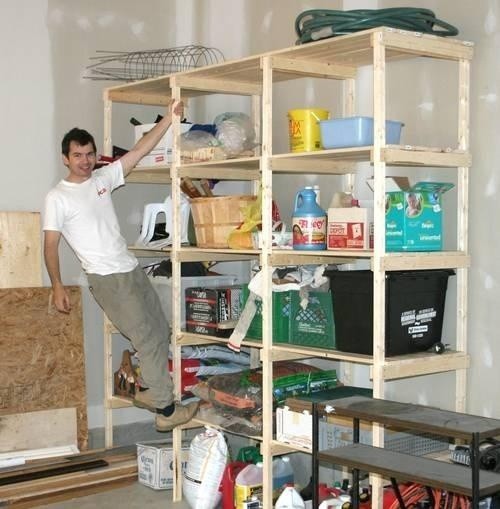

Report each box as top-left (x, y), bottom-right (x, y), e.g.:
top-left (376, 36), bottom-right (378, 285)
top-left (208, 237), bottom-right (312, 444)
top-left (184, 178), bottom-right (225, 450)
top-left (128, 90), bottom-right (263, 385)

top-left (322, 267), bottom-right (457, 358)
top-left (277, 406), bottom-right (316, 452)
top-left (184, 283), bottom-right (241, 338)
top-left (325, 200), bottom-right (377, 252)
top-left (132, 122), bottom-right (195, 166)
top-left (365, 176), bottom-right (454, 252)
top-left (134, 436), bottom-right (197, 491)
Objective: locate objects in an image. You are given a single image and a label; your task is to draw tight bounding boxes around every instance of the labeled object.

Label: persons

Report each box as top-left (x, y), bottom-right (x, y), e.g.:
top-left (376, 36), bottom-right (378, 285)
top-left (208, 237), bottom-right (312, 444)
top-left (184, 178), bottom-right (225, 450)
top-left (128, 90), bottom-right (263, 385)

top-left (43, 98), bottom-right (200, 433)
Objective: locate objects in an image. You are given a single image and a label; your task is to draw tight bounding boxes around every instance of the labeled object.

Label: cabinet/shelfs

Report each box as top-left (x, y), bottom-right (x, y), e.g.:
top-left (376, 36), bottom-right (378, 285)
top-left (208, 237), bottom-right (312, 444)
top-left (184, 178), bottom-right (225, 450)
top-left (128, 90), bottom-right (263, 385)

top-left (100, 63), bottom-right (262, 505)
top-left (265, 32), bottom-right (473, 508)
top-left (309, 394), bottom-right (500, 508)
top-left (174, 26), bottom-right (356, 508)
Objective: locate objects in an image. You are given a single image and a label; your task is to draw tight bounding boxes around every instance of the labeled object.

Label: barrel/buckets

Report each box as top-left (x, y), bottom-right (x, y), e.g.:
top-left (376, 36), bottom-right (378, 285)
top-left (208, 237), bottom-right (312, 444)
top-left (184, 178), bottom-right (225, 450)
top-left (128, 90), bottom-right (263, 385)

top-left (285, 108), bottom-right (331, 154)
top-left (221, 442), bottom-right (353, 509)
top-left (330, 190), bottom-right (354, 209)
top-left (291, 186), bottom-right (328, 251)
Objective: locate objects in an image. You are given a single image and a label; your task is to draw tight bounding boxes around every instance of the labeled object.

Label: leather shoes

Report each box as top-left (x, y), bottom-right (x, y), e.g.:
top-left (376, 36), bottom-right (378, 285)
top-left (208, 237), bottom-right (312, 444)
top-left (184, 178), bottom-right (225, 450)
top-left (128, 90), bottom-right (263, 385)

top-left (155, 397), bottom-right (198, 432)
top-left (133, 385), bottom-right (157, 414)
